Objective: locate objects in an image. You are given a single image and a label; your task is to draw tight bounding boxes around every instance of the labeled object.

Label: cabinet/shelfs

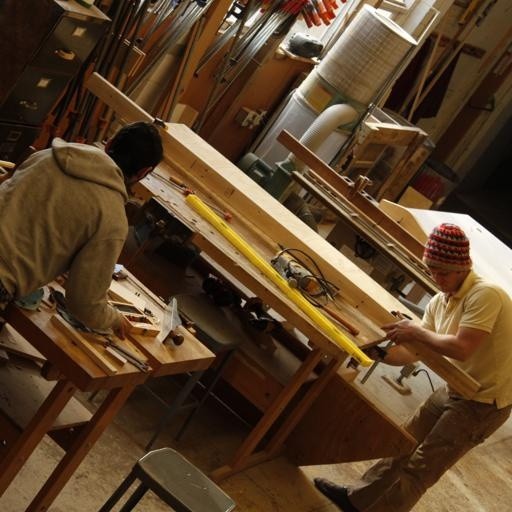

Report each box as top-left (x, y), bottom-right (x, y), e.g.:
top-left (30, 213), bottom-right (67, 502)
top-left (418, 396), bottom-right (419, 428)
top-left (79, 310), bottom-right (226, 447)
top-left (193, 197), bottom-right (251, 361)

top-left (0, 1), bottom-right (110, 158)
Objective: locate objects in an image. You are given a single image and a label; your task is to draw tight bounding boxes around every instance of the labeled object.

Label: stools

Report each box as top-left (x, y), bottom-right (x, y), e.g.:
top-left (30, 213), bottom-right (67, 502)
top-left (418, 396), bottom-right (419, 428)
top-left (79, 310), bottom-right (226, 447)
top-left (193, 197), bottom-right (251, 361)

top-left (94, 290), bottom-right (244, 511)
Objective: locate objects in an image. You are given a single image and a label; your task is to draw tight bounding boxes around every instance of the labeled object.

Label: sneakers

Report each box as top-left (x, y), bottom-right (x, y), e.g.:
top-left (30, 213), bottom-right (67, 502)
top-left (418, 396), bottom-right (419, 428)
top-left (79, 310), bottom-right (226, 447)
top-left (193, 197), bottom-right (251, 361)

top-left (313, 476), bottom-right (357, 512)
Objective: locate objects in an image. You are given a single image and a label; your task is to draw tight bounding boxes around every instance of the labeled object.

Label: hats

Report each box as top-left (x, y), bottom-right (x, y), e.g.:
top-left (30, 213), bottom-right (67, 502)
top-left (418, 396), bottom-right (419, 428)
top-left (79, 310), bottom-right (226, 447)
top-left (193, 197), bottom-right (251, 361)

top-left (421, 220), bottom-right (475, 273)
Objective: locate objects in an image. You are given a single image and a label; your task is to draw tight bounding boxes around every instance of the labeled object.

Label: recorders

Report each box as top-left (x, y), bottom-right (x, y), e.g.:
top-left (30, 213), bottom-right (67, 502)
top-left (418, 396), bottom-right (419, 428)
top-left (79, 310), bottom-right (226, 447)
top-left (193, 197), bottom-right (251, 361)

top-left (290, 32), bottom-right (323, 58)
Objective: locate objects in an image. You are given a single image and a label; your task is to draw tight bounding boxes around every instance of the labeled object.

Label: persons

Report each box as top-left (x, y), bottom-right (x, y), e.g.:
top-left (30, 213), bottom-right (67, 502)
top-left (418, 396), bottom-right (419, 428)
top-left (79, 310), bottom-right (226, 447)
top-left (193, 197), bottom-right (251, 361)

top-left (0, 122), bottom-right (162, 341)
top-left (314, 222), bottom-right (512, 512)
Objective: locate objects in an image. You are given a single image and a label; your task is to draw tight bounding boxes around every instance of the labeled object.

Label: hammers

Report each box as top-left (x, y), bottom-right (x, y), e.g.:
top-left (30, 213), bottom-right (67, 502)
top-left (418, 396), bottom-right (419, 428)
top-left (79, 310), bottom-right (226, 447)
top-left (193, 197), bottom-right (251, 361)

top-left (288, 275), bottom-right (359, 336)
